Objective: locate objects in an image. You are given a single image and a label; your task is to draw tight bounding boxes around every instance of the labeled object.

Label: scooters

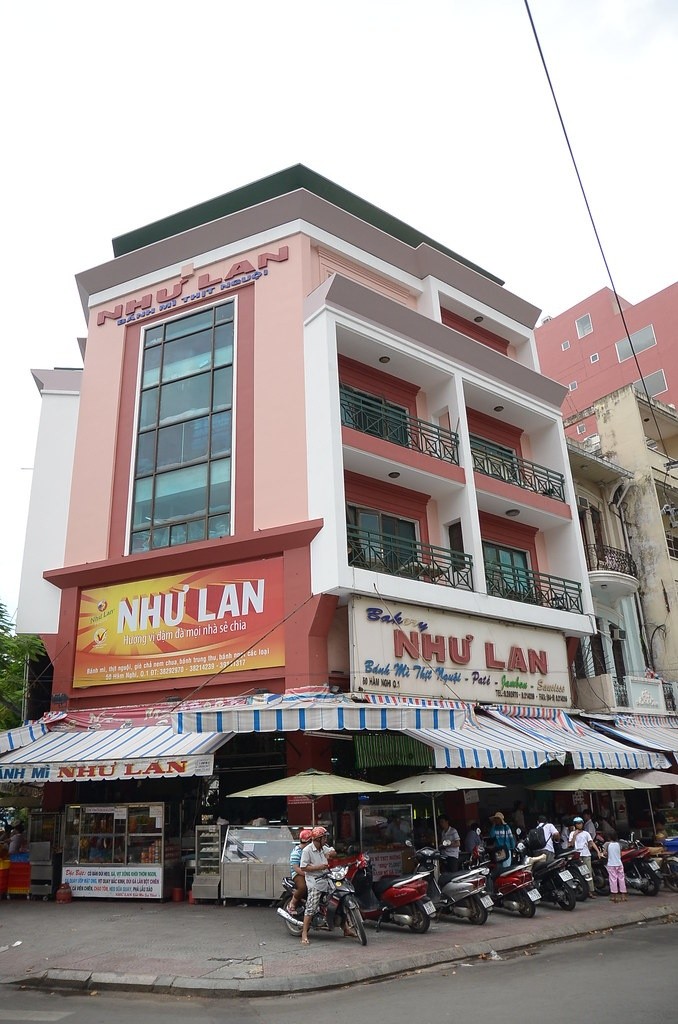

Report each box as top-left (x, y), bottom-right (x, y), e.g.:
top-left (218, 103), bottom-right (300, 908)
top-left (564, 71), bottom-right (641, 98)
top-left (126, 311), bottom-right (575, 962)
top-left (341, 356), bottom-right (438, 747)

top-left (405, 838), bottom-right (494, 926)
top-left (342, 848), bottom-right (437, 934)
top-left (460, 827), bottom-right (543, 919)
top-left (509, 827), bottom-right (678, 911)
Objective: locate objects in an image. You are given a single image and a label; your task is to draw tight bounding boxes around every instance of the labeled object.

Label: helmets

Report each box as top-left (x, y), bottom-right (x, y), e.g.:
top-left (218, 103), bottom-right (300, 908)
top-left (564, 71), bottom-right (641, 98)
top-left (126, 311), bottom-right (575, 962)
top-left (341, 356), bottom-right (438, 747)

top-left (313, 826), bottom-right (330, 841)
top-left (300, 830), bottom-right (312, 842)
top-left (573, 817), bottom-right (584, 825)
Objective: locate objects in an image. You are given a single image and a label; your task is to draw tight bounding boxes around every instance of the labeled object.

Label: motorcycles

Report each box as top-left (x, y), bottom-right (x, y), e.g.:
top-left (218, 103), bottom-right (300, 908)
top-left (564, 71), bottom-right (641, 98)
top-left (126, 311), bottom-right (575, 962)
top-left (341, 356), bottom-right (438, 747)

top-left (275, 860), bottom-right (367, 946)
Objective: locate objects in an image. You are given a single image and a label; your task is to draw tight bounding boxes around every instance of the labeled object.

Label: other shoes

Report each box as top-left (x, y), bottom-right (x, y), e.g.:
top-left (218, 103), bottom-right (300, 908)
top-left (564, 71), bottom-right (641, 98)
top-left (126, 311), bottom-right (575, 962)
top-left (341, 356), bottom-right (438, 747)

top-left (609, 897), bottom-right (616, 902)
top-left (588, 893), bottom-right (597, 899)
top-left (287, 902), bottom-right (297, 915)
top-left (622, 898), bottom-right (627, 902)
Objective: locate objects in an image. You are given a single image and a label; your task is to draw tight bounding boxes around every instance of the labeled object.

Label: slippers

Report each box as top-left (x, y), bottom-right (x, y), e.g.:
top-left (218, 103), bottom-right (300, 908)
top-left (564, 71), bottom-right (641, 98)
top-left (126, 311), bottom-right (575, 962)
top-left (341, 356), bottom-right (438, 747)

top-left (299, 937), bottom-right (310, 946)
top-left (343, 929), bottom-right (358, 937)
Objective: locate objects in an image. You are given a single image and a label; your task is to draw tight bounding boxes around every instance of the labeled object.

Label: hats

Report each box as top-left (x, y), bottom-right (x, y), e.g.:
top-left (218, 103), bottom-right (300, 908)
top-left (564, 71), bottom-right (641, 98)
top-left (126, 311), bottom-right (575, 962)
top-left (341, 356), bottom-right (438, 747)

top-left (489, 813), bottom-right (507, 825)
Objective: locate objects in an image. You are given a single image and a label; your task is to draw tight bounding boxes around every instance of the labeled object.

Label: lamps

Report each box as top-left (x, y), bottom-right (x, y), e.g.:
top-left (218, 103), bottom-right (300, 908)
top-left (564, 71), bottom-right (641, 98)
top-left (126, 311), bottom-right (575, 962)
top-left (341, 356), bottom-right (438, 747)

top-left (52, 692), bottom-right (67, 704)
top-left (331, 685), bottom-right (339, 693)
top-left (506, 509), bottom-right (520, 516)
top-left (601, 585), bottom-right (607, 589)
top-left (582, 466), bottom-right (587, 470)
top-left (389, 472), bottom-right (400, 478)
top-left (474, 317), bottom-right (483, 322)
top-left (494, 406), bottom-right (504, 411)
top-left (379, 356), bottom-right (390, 363)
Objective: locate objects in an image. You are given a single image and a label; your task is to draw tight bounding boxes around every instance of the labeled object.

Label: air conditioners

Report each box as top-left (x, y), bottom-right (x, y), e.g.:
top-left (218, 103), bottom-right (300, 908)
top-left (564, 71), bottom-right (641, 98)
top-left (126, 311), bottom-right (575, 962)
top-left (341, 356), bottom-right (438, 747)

top-left (611, 629), bottom-right (626, 640)
top-left (576, 495), bottom-right (589, 510)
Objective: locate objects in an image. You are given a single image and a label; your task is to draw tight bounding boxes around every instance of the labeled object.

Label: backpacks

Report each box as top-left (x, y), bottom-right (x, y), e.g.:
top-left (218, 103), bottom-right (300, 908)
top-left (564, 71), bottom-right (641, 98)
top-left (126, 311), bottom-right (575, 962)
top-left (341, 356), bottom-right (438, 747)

top-left (529, 823), bottom-right (553, 850)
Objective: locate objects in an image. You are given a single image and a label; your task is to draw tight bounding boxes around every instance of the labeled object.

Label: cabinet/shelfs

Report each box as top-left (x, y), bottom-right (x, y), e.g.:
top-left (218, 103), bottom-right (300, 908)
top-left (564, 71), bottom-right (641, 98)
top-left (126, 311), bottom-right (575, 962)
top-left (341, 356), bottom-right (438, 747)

top-left (196, 824), bottom-right (226, 877)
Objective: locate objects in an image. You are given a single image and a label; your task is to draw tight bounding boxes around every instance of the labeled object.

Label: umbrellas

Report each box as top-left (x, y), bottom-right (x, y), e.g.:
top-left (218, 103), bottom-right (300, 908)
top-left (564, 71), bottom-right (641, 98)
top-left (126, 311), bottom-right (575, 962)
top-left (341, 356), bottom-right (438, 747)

top-left (226, 764), bottom-right (399, 830)
top-left (525, 770), bottom-right (662, 814)
top-left (623, 769), bottom-right (678, 836)
top-left (0, 796), bottom-right (43, 812)
top-left (382, 766), bottom-right (507, 885)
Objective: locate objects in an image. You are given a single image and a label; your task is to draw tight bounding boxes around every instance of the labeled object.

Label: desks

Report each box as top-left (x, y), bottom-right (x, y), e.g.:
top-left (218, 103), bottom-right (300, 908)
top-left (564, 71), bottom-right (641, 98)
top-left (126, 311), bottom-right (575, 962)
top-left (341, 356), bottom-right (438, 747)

top-left (399, 562), bottom-right (448, 581)
top-left (184, 866), bottom-right (195, 901)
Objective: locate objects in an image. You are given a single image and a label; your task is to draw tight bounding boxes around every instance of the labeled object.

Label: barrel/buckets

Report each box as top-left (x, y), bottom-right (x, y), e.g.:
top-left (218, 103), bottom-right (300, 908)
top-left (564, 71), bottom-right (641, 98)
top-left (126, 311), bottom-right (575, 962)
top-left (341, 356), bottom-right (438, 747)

top-left (172, 887), bottom-right (184, 902)
top-left (189, 890), bottom-right (195, 905)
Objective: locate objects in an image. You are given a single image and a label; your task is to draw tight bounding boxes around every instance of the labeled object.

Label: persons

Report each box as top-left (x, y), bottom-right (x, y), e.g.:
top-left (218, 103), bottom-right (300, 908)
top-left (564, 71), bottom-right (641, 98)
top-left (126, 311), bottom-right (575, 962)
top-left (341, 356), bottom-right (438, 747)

top-left (513, 800), bottom-right (629, 903)
top-left (0, 819), bottom-right (25, 853)
top-left (384, 810), bottom-right (461, 873)
top-left (286, 827), bottom-right (337, 944)
top-left (464, 811), bottom-right (515, 867)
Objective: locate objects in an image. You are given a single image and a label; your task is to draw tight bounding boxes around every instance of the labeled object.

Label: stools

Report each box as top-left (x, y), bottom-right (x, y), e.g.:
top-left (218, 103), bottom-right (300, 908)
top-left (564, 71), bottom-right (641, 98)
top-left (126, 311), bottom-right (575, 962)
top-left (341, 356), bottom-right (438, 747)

top-left (550, 596), bottom-right (564, 608)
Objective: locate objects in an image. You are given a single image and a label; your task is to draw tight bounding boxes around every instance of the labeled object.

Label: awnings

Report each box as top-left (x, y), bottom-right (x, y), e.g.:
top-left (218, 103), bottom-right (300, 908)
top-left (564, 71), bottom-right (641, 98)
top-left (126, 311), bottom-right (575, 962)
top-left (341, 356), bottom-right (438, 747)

top-left (0, 782), bottom-right (46, 798)
top-left (0, 723), bottom-right (49, 753)
top-left (479, 703), bottom-right (673, 770)
top-left (365, 692), bottom-right (564, 769)
top-left (589, 712), bottom-right (678, 764)
top-left (0, 694), bottom-right (252, 782)
top-left (170, 701), bottom-right (466, 734)
